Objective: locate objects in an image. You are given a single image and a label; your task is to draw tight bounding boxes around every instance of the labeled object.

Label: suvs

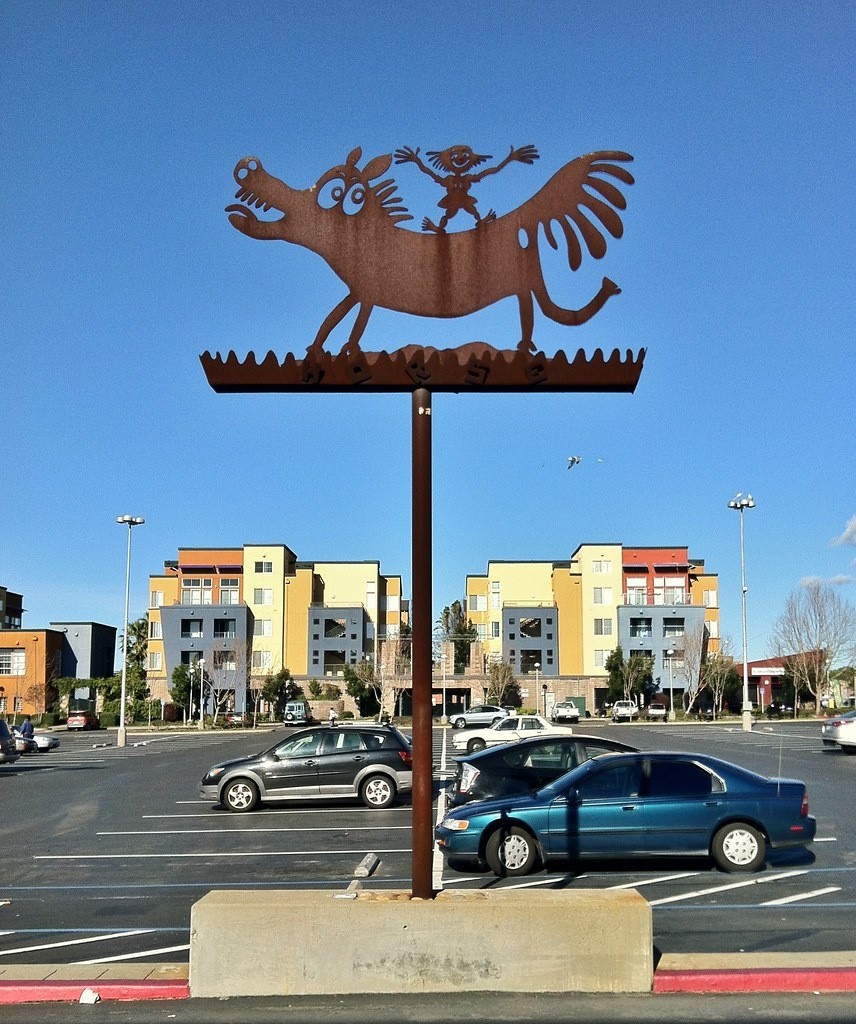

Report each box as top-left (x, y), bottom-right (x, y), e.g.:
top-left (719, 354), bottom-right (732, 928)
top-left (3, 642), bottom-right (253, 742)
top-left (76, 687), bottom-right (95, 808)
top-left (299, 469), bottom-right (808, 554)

top-left (66, 709), bottom-right (100, 731)
top-left (282, 700), bottom-right (315, 727)
top-left (0, 718), bottom-right (21, 763)
top-left (196, 721), bottom-right (437, 813)
top-left (449, 704), bottom-right (511, 729)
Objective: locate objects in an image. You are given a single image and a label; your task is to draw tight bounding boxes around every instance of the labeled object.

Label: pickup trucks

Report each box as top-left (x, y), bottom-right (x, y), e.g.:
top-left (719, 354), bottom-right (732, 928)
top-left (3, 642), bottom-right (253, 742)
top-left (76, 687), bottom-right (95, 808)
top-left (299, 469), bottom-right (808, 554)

top-left (551, 700), bottom-right (580, 724)
top-left (611, 699), bottom-right (639, 723)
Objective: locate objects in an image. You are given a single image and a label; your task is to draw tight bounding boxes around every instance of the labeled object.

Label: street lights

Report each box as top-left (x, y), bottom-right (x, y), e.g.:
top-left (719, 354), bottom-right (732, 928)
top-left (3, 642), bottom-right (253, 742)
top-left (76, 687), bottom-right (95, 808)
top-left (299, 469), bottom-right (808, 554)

top-left (197, 659), bottom-right (206, 731)
top-left (117, 513), bottom-right (146, 747)
top-left (440, 654), bottom-right (448, 725)
top-left (727, 491), bottom-right (757, 731)
top-left (666, 649), bottom-right (675, 722)
top-left (534, 663), bottom-right (540, 717)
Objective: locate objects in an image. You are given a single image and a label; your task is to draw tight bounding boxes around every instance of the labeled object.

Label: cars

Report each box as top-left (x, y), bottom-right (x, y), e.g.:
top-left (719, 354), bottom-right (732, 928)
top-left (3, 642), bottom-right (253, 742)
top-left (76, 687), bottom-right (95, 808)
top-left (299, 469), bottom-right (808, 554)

top-left (451, 715), bottom-right (573, 754)
top-left (444, 733), bottom-right (663, 805)
top-left (766, 700), bottom-right (799, 721)
top-left (433, 751), bottom-right (817, 877)
top-left (646, 703), bottom-right (667, 723)
top-left (504, 705), bottom-right (518, 718)
top-left (822, 708), bottom-right (856, 754)
top-left (8, 724), bottom-right (61, 753)
top-left (226, 712), bottom-right (254, 728)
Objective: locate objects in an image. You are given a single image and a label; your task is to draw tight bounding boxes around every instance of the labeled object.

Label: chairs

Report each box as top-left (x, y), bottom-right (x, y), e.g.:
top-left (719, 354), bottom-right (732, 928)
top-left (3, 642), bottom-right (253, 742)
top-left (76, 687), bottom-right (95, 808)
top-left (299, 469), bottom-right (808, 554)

top-left (561, 745), bottom-right (571, 768)
top-left (567, 748), bottom-right (586, 768)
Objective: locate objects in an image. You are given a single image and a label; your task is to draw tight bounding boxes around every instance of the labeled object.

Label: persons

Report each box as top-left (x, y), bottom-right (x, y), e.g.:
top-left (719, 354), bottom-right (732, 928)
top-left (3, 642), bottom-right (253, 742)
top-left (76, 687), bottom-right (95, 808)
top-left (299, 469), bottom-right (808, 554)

top-left (19, 715), bottom-right (35, 753)
top-left (328, 706), bottom-right (338, 727)
